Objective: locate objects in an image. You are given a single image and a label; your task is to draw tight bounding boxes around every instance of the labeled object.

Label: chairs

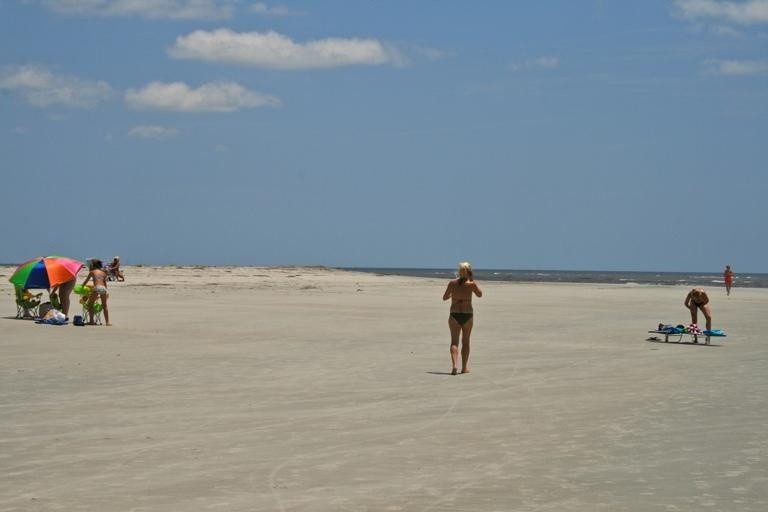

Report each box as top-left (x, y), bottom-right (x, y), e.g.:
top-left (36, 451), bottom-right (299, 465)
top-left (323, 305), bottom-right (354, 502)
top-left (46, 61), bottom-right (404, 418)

top-left (16, 297), bottom-right (41, 319)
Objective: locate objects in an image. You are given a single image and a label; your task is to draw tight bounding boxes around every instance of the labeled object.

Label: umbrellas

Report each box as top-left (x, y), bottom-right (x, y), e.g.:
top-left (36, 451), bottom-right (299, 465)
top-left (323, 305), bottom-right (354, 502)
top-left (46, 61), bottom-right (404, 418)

top-left (8, 254), bottom-right (85, 290)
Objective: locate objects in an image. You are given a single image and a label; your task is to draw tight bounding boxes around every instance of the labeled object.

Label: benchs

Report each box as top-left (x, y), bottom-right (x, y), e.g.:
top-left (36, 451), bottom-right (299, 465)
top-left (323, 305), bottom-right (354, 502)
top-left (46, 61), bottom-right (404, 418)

top-left (648, 330), bottom-right (726, 345)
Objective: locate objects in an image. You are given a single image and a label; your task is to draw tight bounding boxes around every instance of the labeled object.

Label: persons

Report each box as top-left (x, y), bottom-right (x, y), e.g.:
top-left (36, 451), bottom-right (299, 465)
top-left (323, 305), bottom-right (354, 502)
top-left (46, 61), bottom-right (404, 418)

top-left (684, 286), bottom-right (711, 345)
top-left (110, 256), bottom-right (125, 282)
top-left (441, 262), bottom-right (482, 374)
top-left (81, 259), bottom-right (113, 325)
top-left (723, 264), bottom-right (734, 296)
top-left (47, 276), bottom-right (76, 321)
top-left (21, 288), bottom-right (32, 318)
